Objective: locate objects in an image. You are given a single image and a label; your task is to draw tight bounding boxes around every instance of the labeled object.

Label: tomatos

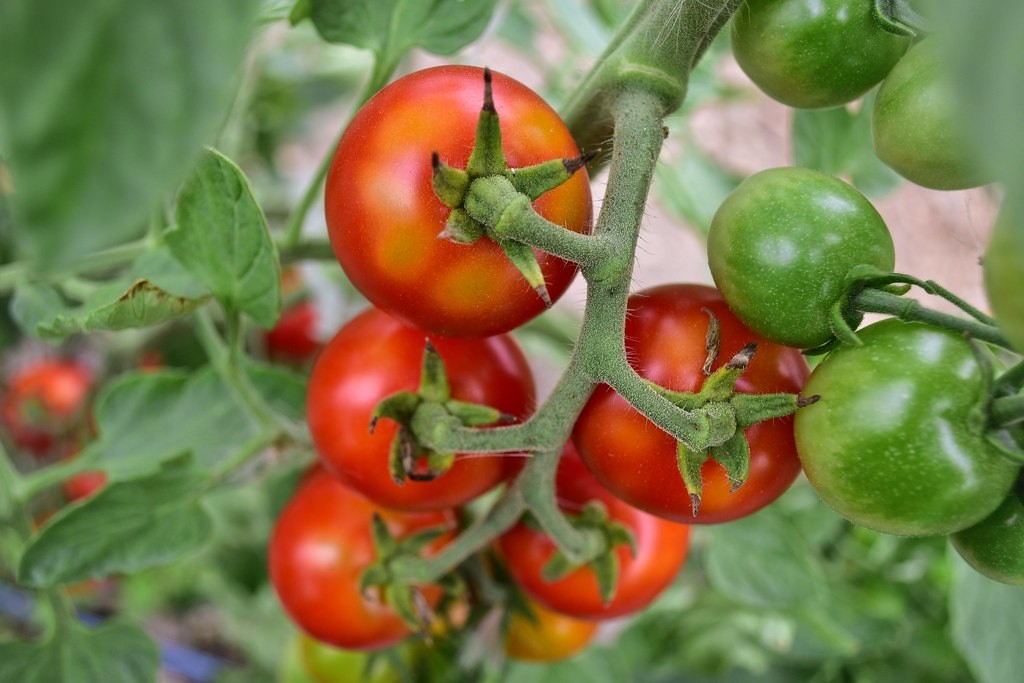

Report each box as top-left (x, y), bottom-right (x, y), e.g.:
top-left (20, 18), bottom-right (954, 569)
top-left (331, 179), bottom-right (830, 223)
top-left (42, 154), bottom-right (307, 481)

top-left (0, 0), bottom-right (1024, 683)
